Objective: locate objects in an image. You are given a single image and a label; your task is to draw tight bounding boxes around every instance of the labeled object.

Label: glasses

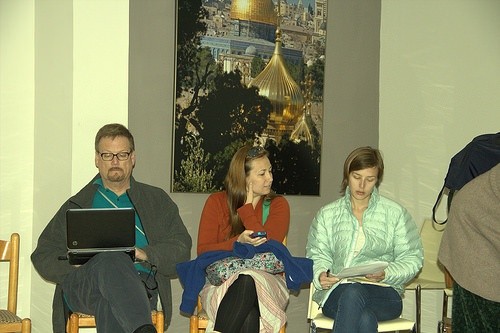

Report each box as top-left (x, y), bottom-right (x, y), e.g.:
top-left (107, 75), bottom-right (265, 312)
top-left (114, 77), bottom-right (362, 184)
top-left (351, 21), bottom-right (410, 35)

top-left (245, 146), bottom-right (264, 158)
top-left (96, 150), bottom-right (133, 161)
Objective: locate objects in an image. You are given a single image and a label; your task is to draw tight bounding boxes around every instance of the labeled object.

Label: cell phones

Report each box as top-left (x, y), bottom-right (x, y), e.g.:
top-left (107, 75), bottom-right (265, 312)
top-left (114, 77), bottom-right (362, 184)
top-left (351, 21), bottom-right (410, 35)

top-left (250, 231), bottom-right (267, 238)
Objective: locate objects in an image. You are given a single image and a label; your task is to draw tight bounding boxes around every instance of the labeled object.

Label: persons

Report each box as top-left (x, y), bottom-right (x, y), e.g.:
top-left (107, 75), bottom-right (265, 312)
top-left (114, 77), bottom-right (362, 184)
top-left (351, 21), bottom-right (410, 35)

top-left (438, 162), bottom-right (500, 333)
top-left (197, 145), bottom-right (290, 333)
top-left (306, 147), bottom-right (425, 333)
top-left (30, 124), bottom-right (192, 333)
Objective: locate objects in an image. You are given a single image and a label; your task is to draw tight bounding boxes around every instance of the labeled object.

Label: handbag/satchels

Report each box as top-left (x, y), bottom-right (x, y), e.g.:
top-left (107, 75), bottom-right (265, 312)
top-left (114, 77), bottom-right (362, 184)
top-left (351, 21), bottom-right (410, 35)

top-left (206, 252), bottom-right (285, 286)
top-left (431, 132), bottom-right (500, 224)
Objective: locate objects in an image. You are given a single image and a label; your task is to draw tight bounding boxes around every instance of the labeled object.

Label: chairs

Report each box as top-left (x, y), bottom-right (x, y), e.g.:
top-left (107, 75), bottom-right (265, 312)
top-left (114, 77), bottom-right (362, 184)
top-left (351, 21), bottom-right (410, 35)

top-left (307, 280), bottom-right (421, 333)
top-left (0, 233), bottom-right (31, 333)
top-left (190, 235), bottom-right (286, 333)
top-left (68, 310), bottom-right (164, 333)
top-left (438, 289), bottom-right (454, 333)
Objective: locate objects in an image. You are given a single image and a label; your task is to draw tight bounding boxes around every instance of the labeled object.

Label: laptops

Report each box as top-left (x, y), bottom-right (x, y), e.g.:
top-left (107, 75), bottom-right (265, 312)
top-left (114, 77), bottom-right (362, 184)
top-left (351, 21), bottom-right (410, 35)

top-left (64, 207), bottom-right (137, 265)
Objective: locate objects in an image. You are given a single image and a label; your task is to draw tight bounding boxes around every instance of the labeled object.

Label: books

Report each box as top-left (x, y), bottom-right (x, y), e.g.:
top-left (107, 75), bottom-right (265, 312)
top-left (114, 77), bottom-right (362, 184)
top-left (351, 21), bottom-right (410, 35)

top-left (350, 277), bottom-right (388, 287)
